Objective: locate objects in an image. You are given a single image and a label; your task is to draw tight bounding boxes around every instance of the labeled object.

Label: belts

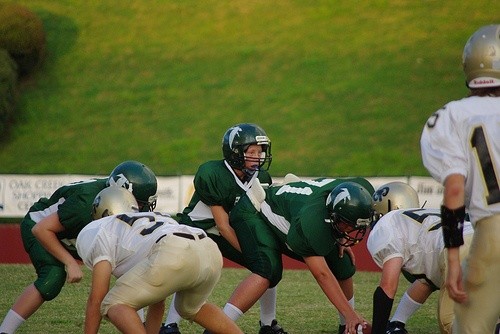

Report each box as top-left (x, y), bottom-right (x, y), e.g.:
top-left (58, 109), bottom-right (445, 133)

top-left (156, 231), bottom-right (207, 242)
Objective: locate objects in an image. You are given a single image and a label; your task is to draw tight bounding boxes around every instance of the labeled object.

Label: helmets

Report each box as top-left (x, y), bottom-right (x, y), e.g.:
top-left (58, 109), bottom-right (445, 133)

top-left (370, 183), bottom-right (419, 221)
top-left (92, 187), bottom-right (139, 219)
top-left (222, 123), bottom-right (271, 175)
top-left (106, 161), bottom-right (159, 213)
top-left (326, 181), bottom-right (375, 247)
top-left (463, 23), bottom-right (500, 88)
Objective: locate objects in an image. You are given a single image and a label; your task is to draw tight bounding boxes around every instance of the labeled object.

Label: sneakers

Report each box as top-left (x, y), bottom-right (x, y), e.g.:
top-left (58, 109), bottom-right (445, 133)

top-left (258, 319), bottom-right (288, 334)
top-left (387, 320), bottom-right (408, 334)
top-left (159, 322), bottom-right (180, 334)
top-left (339, 324), bottom-right (346, 334)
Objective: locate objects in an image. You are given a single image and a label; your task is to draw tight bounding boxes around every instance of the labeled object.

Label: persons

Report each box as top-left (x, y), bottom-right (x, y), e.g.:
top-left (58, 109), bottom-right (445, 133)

top-left (202, 177), bottom-right (376, 334)
top-left (0, 160), bottom-right (157, 334)
top-left (367, 182), bottom-right (476, 334)
top-left (420, 23), bottom-right (500, 334)
top-left (75, 186), bottom-right (246, 334)
top-left (159, 122), bottom-right (287, 334)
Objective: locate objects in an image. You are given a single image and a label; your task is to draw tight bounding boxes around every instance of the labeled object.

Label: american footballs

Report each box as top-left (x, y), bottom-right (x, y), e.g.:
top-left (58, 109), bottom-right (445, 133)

top-left (345, 321), bottom-right (372, 334)
